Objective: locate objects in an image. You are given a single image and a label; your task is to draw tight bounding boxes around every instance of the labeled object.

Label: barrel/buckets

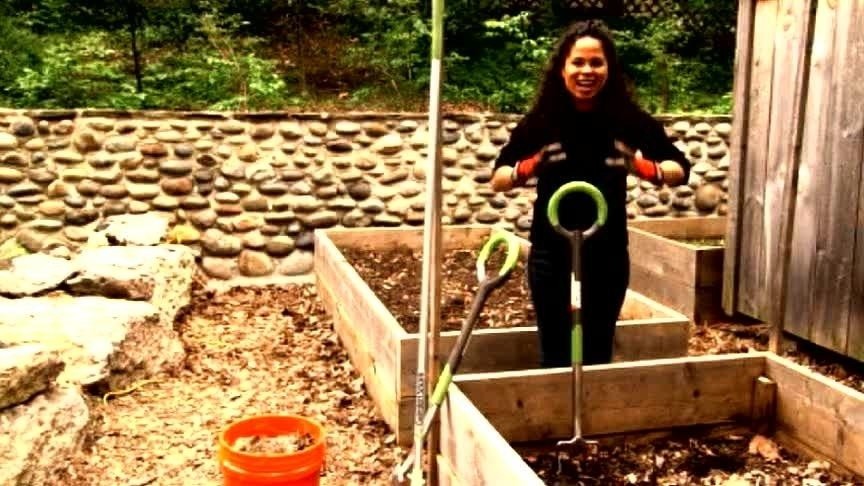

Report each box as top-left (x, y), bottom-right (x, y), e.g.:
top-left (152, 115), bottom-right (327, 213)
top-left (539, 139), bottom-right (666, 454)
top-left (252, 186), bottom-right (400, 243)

top-left (219, 415), bottom-right (327, 486)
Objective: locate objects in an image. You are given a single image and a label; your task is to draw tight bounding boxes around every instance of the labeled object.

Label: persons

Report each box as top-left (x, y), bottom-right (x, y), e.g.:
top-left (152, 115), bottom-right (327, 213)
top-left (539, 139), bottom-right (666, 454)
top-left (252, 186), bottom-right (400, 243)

top-left (490, 17), bottom-right (691, 367)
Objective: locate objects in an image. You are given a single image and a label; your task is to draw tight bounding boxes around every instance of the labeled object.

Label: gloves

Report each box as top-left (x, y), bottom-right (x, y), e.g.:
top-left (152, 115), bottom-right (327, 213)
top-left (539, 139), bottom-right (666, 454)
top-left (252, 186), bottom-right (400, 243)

top-left (604, 142), bottom-right (663, 186)
top-left (512, 144), bottom-right (567, 187)
